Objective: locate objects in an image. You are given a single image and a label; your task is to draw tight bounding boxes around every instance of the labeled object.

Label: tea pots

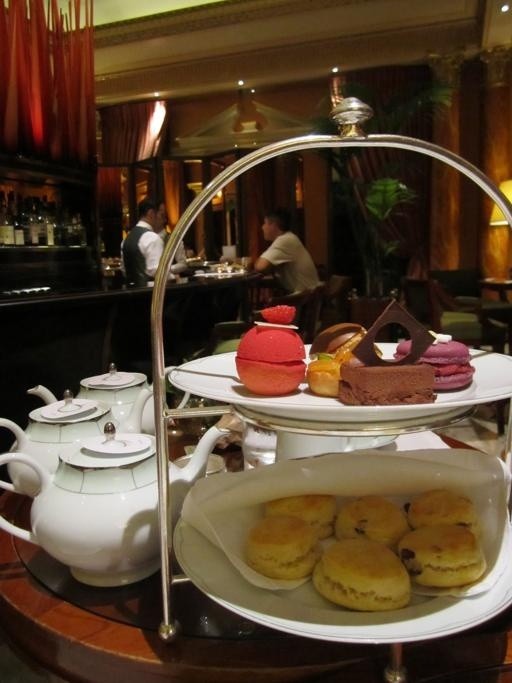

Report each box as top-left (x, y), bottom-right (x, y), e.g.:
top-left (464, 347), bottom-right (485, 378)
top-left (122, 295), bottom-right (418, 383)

top-left (2, 387), bottom-right (156, 497)
top-left (25, 361), bottom-right (192, 433)
top-left (1, 421), bottom-right (232, 590)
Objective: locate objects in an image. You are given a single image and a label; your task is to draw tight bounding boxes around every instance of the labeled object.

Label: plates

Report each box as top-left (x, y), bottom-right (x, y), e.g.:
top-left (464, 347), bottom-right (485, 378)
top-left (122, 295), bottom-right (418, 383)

top-left (167, 340), bottom-right (512, 424)
top-left (172, 450), bottom-right (511, 644)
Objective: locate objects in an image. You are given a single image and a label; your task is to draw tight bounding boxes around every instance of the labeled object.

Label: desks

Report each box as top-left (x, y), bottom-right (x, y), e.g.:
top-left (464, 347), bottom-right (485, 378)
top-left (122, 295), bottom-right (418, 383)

top-left (480, 279), bottom-right (512, 302)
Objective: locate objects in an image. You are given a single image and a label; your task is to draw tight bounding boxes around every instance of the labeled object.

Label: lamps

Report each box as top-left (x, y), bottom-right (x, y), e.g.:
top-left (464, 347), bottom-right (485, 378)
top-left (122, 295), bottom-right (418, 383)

top-left (488, 180), bottom-right (512, 226)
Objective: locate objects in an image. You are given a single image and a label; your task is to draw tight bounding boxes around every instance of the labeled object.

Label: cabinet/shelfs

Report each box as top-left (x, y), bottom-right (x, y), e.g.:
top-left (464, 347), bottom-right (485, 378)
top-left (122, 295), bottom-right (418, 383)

top-left (1, 152), bottom-right (105, 290)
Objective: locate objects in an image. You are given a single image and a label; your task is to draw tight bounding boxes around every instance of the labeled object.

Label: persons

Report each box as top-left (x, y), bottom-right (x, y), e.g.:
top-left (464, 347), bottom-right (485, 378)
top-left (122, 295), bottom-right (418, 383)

top-left (156, 210), bottom-right (190, 274)
top-left (236, 205), bottom-right (323, 345)
top-left (116, 195), bottom-right (181, 284)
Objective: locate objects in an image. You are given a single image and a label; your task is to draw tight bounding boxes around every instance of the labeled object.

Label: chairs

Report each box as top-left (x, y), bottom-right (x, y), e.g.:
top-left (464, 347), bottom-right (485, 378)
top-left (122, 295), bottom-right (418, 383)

top-left (422, 271), bottom-right (512, 317)
top-left (268, 283), bottom-right (326, 345)
top-left (404, 280), bottom-right (482, 350)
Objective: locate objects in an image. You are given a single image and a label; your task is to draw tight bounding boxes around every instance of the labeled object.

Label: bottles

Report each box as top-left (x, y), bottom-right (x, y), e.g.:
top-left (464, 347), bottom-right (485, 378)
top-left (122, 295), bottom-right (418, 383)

top-left (0, 191), bottom-right (6, 245)
top-left (23, 198), bottom-right (31, 246)
top-left (30, 198), bottom-right (39, 245)
top-left (13, 192), bottom-right (25, 246)
top-left (46, 201), bottom-right (55, 245)
top-left (37, 198), bottom-right (47, 245)
top-left (4, 191), bottom-right (15, 246)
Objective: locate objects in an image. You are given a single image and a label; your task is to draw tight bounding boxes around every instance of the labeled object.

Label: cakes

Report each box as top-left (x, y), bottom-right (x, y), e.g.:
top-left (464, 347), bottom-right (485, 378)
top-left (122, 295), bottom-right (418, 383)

top-left (396, 331), bottom-right (475, 391)
top-left (314, 539), bottom-right (411, 611)
top-left (246, 515), bottom-right (322, 580)
top-left (334, 498), bottom-right (408, 549)
top-left (408, 492), bottom-right (476, 529)
top-left (339, 300), bottom-right (436, 405)
top-left (264, 494), bottom-right (336, 539)
top-left (309, 323), bottom-right (382, 396)
top-left (237, 305), bottom-right (306, 395)
top-left (400, 523), bottom-right (486, 586)
